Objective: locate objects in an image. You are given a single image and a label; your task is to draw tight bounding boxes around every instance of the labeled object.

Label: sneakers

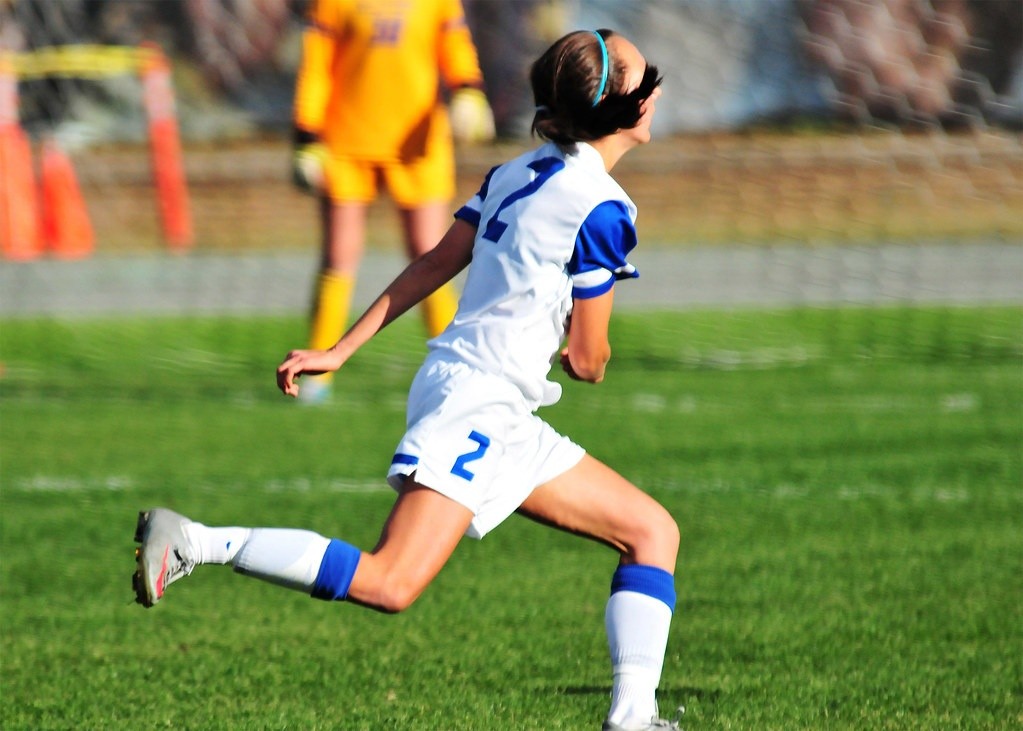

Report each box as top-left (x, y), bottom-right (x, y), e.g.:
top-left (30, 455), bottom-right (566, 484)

top-left (601, 718), bottom-right (681, 731)
top-left (134, 503), bottom-right (197, 609)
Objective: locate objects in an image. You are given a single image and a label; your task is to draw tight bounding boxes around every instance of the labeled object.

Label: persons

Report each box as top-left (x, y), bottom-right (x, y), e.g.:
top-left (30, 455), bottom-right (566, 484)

top-left (288, 3), bottom-right (497, 417)
top-left (134, 31), bottom-right (694, 731)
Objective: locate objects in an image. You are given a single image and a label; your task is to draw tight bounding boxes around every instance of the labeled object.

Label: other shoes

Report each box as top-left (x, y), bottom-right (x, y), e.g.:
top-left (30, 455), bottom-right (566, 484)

top-left (296, 379), bottom-right (332, 404)
